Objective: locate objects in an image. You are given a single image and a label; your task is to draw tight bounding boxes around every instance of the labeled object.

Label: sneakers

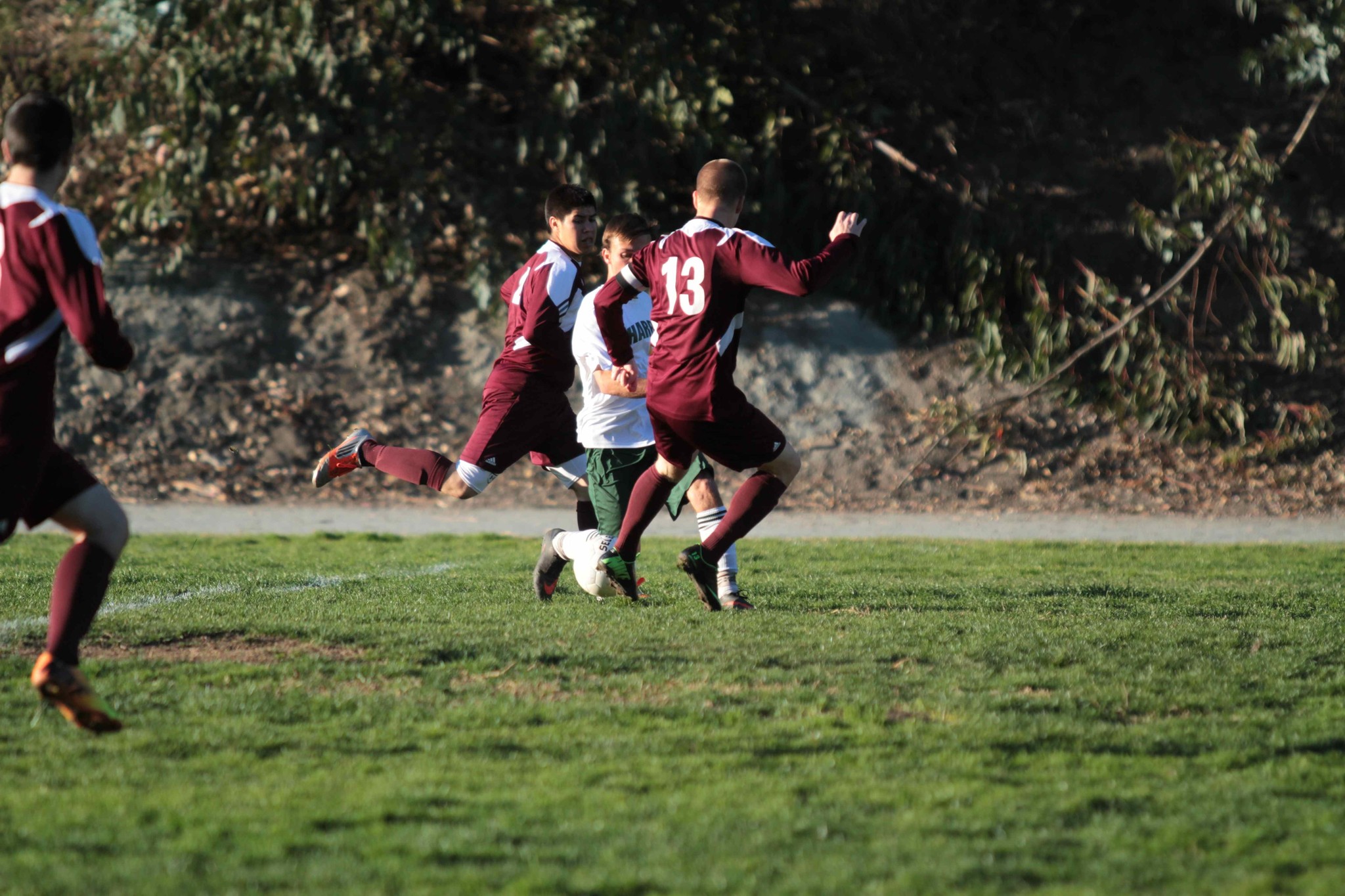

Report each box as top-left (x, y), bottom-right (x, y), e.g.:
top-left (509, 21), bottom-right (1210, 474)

top-left (719, 589), bottom-right (754, 609)
top-left (678, 543), bottom-right (721, 611)
top-left (596, 547), bottom-right (640, 602)
top-left (636, 577), bottom-right (648, 598)
top-left (31, 651), bottom-right (123, 736)
top-left (533, 528), bottom-right (571, 604)
top-left (312, 428), bottom-right (378, 490)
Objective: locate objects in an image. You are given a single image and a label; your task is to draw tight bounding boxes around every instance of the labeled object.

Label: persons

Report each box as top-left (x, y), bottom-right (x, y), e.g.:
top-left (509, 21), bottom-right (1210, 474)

top-left (0, 93), bottom-right (134, 733)
top-left (311, 184), bottom-right (646, 587)
top-left (534, 212), bottom-right (755, 613)
top-left (595, 159), bottom-right (867, 612)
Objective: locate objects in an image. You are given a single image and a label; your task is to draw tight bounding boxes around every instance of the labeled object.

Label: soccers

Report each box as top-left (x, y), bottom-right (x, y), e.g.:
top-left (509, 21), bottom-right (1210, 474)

top-left (573, 535), bottom-right (618, 599)
